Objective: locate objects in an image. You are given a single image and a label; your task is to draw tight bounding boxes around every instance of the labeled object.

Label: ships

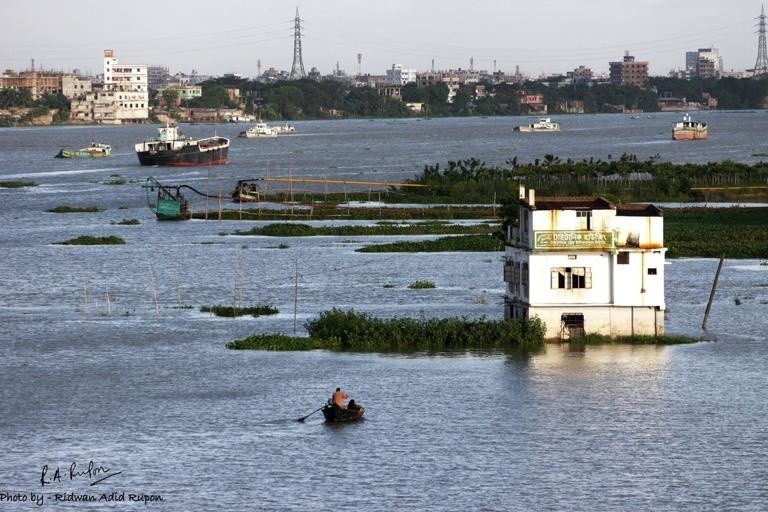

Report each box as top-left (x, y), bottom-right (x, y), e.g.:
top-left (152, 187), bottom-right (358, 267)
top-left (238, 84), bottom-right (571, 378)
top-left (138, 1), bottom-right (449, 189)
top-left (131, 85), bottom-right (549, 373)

top-left (239, 120), bottom-right (280, 138)
top-left (512, 119), bottom-right (560, 133)
top-left (135, 117), bottom-right (230, 168)
top-left (671, 112), bottom-right (707, 139)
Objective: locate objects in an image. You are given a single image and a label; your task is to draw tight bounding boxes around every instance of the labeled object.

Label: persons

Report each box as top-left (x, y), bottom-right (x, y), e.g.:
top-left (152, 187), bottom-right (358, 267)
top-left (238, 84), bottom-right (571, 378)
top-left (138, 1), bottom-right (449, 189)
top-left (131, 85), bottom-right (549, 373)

top-left (346, 399), bottom-right (365, 412)
top-left (332, 387), bottom-right (350, 409)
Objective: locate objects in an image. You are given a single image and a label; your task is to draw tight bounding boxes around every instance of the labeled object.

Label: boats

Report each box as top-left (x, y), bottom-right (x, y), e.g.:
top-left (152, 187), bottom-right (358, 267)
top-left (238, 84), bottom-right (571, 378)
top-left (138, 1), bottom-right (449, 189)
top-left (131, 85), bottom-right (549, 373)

top-left (58, 137), bottom-right (112, 158)
top-left (232, 183), bottom-right (261, 203)
top-left (273, 124), bottom-right (296, 135)
top-left (322, 400), bottom-right (365, 423)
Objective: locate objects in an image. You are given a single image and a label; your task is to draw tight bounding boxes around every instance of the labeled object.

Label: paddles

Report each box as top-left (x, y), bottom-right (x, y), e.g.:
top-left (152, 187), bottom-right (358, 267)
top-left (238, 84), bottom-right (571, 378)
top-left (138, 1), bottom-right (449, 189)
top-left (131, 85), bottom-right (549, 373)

top-left (298, 403), bottom-right (330, 422)
